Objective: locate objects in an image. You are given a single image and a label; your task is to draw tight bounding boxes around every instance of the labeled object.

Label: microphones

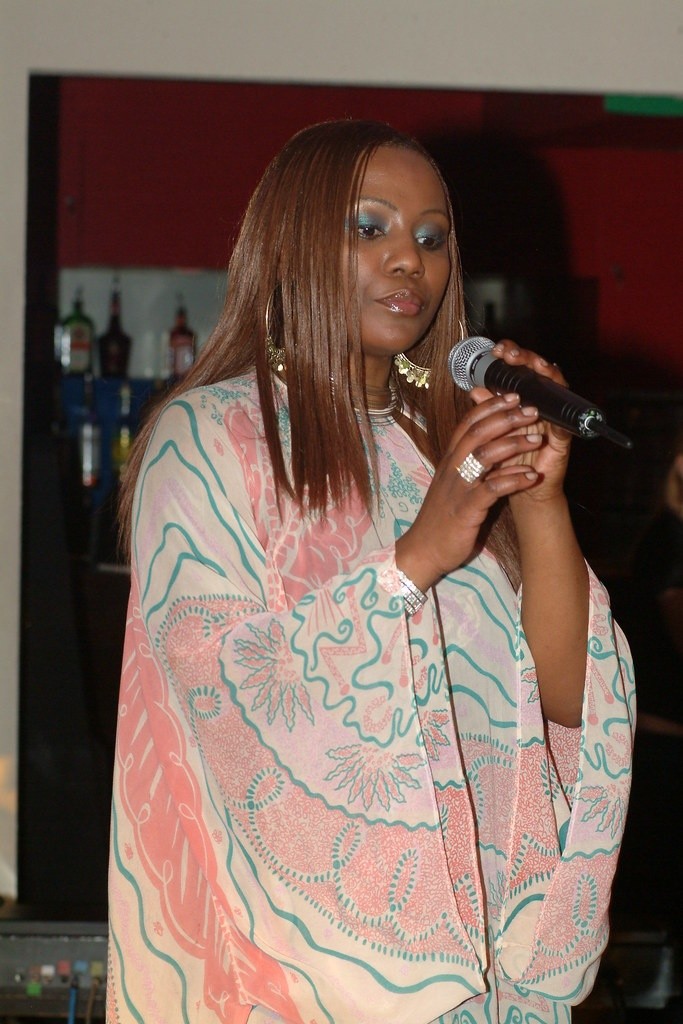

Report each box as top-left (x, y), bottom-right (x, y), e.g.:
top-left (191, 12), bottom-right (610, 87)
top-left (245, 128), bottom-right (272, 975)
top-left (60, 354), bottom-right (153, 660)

top-left (446, 336), bottom-right (634, 449)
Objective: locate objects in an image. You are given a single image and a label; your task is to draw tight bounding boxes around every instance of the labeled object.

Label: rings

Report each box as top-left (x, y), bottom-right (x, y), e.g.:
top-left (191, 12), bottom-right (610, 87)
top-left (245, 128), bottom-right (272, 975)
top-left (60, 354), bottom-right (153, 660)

top-left (458, 454), bottom-right (485, 484)
top-left (554, 363), bottom-right (559, 368)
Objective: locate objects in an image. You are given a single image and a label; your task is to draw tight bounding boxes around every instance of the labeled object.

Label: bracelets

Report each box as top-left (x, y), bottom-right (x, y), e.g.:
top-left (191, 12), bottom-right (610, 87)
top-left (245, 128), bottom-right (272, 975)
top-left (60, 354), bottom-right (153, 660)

top-left (397, 571), bottom-right (426, 614)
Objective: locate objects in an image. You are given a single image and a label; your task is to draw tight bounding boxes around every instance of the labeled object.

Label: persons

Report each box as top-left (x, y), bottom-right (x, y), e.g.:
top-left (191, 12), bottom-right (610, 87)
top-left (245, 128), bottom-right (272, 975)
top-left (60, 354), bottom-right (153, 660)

top-left (103, 123), bottom-right (637, 1023)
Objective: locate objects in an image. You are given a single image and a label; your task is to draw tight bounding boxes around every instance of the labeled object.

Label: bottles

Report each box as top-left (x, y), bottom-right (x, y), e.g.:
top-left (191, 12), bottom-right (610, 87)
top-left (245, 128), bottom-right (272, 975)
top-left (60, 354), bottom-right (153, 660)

top-left (58, 287), bottom-right (93, 380)
top-left (169, 306), bottom-right (196, 378)
top-left (97, 277), bottom-right (131, 379)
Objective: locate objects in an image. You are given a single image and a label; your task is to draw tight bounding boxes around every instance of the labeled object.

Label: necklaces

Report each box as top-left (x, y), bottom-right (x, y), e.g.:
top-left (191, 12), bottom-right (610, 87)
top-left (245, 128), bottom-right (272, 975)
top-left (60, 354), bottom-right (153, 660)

top-left (278, 366), bottom-right (398, 415)
